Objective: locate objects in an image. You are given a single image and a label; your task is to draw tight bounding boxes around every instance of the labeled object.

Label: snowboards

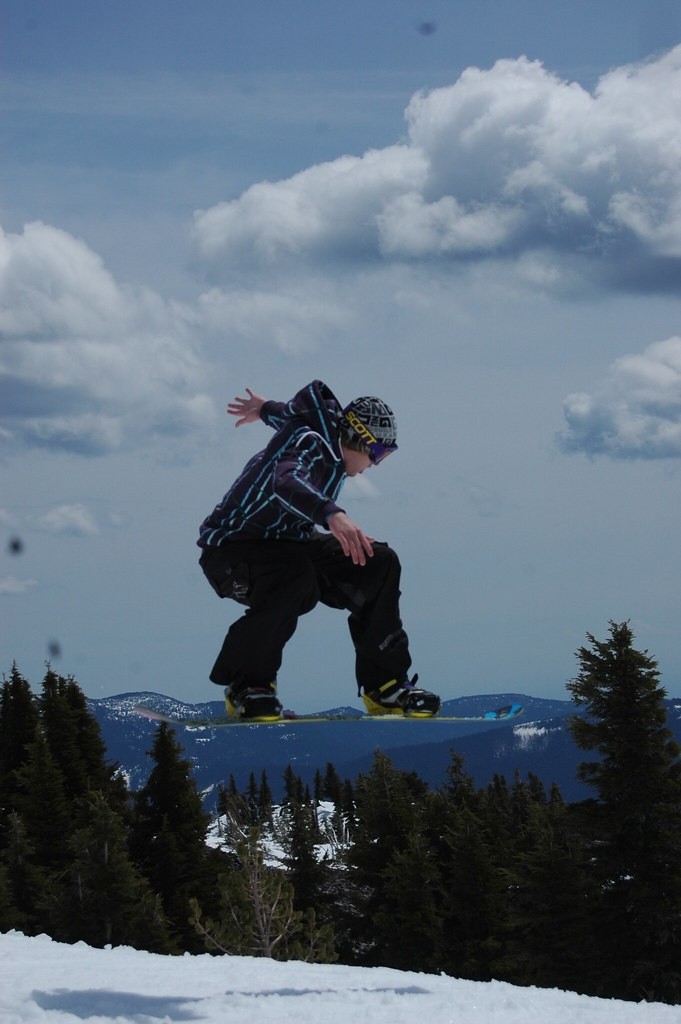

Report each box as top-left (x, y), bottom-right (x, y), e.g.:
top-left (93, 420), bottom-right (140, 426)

top-left (132, 702), bottom-right (526, 729)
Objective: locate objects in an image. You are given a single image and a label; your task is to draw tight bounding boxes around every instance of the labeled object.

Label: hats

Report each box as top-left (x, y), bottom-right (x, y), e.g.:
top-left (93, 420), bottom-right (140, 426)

top-left (341, 396), bottom-right (397, 447)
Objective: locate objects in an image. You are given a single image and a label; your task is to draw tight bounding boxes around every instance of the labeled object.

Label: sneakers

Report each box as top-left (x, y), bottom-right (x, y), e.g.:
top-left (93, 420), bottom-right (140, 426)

top-left (224, 682), bottom-right (283, 721)
top-left (361, 674), bottom-right (441, 718)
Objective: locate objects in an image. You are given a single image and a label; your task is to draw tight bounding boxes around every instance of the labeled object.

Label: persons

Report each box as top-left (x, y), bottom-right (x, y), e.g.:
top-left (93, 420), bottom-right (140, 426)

top-left (198, 379), bottom-right (441, 720)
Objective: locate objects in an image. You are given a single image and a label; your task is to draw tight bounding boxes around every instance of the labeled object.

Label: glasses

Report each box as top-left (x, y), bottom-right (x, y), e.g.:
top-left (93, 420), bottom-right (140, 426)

top-left (367, 444), bottom-right (398, 465)
